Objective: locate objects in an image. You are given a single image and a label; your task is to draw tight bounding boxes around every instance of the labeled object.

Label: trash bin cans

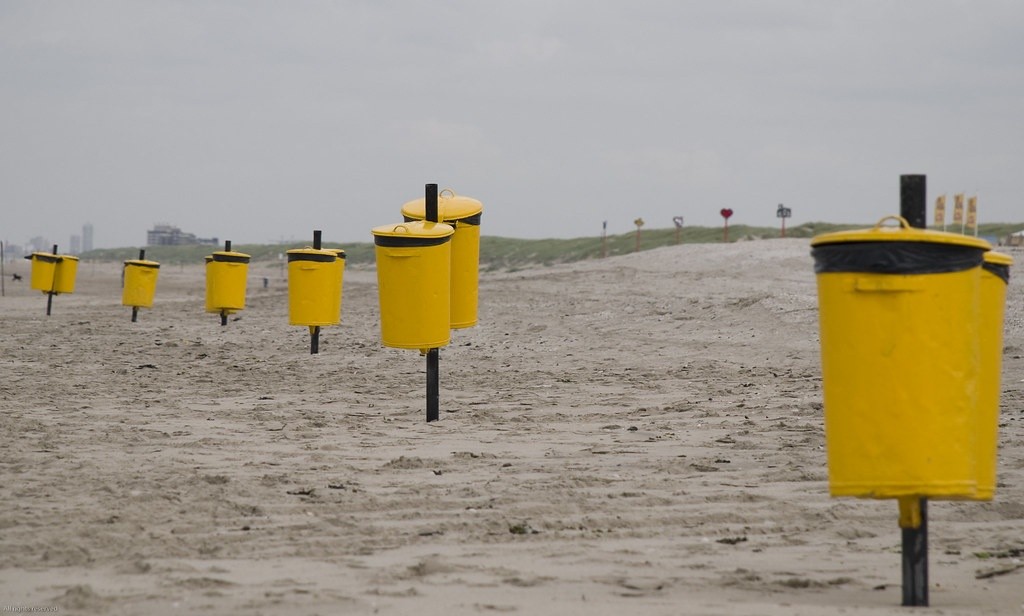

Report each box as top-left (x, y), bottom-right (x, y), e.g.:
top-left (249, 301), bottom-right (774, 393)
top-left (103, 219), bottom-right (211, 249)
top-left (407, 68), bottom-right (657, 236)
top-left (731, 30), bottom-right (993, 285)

top-left (810, 214), bottom-right (1015, 499)
top-left (401, 188), bottom-right (483, 328)
top-left (284, 246), bottom-right (348, 327)
top-left (28, 252), bottom-right (79, 293)
top-left (205, 250), bottom-right (252, 312)
top-left (121, 259), bottom-right (159, 307)
top-left (371, 219), bottom-right (456, 347)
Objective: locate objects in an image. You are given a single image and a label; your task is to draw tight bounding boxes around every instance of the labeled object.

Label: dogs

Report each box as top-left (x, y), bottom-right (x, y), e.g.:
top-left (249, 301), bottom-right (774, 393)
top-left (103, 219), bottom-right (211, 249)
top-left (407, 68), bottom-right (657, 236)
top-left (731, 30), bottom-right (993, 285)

top-left (10, 272), bottom-right (23, 284)
